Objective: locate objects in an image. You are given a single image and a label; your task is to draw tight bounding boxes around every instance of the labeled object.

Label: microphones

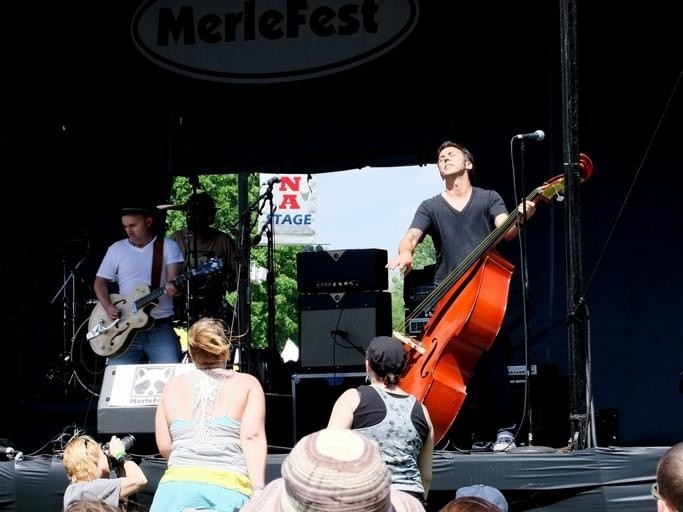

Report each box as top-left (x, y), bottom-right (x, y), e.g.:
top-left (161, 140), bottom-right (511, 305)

top-left (225, 222), bottom-right (241, 240)
top-left (332, 330), bottom-right (350, 337)
top-left (253, 213), bottom-right (274, 246)
top-left (516, 130), bottom-right (545, 142)
top-left (263, 175), bottom-right (282, 185)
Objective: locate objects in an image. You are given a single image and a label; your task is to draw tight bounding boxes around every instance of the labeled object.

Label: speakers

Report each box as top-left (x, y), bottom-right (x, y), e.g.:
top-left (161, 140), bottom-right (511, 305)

top-left (298, 292), bottom-right (392, 373)
top-left (297, 248), bottom-right (389, 293)
top-left (97, 363), bottom-right (195, 455)
top-left (292, 372), bottom-right (371, 445)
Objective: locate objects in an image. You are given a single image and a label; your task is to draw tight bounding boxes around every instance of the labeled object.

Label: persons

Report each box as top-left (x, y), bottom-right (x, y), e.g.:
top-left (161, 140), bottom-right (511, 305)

top-left (385, 141), bottom-right (536, 453)
top-left (326, 335), bottom-right (434, 508)
top-left (149, 317), bottom-right (268, 512)
top-left (170, 193), bottom-right (238, 369)
top-left (93, 207), bottom-right (186, 364)
top-left (655, 442), bottom-right (683, 512)
top-left (439, 496), bottom-right (503, 512)
top-left (63, 435), bottom-right (148, 512)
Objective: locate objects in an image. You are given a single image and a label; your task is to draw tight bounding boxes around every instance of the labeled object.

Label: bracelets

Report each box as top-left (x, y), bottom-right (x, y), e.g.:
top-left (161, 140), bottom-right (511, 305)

top-left (515, 221), bottom-right (527, 229)
top-left (114, 452), bottom-right (132, 466)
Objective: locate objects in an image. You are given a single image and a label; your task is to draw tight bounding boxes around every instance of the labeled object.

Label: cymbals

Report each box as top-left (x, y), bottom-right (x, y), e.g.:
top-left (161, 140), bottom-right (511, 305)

top-left (156, 204), bottom-right (223, 211)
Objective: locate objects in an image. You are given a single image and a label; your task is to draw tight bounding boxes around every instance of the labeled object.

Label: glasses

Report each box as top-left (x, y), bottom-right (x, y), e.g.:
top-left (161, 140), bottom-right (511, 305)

top-left (650, 482), bottom-right (664, 502)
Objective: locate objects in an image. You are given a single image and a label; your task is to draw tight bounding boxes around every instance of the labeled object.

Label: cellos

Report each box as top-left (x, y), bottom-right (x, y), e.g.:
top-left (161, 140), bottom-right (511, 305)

top-left (389, 151), bottom-right (594, 448)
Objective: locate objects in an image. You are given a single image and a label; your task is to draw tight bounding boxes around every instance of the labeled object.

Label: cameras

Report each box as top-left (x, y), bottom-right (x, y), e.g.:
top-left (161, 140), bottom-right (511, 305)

top-left (101, 434), bottom-right (137, 471)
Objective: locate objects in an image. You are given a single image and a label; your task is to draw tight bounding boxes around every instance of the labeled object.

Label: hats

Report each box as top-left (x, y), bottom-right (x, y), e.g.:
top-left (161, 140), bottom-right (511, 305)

top-left (366, 337), bottom-right (405, 376)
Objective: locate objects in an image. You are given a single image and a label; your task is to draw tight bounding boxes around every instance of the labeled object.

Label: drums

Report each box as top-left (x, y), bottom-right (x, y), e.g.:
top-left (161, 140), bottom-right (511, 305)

top-left (71, 315), bottom-right (107, 397)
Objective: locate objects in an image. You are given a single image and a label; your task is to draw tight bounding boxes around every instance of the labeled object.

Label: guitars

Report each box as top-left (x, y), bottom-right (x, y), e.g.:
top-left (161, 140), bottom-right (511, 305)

top-left (86, 256), bottom-right (225, 359)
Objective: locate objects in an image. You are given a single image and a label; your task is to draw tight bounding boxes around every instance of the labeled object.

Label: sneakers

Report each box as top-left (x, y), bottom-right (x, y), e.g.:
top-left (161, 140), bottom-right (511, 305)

top-left (493, 430), bottom-right (515, 451)
